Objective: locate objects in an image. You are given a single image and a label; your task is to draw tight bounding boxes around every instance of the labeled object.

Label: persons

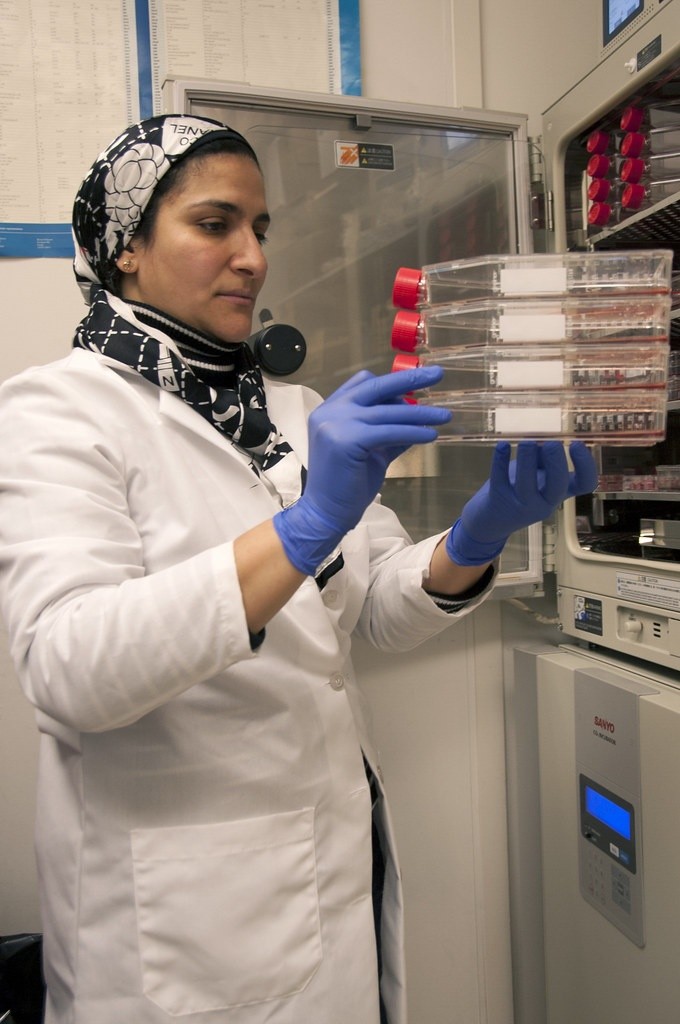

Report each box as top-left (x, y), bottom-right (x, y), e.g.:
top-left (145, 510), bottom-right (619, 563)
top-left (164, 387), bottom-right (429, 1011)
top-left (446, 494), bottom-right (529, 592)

top-left (0, 115), bottom-right (599, 1024)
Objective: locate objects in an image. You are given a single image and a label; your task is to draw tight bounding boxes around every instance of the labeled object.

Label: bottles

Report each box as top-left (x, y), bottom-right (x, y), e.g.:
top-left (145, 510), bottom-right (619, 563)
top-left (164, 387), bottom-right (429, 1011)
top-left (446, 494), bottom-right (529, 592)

top-left (586, 131), bottom-right (620, 153)
top-left (619, 103), bottom-right (680, 132)
top-left (621, 132), bottom-right (680, 158)
top-left (399, 394), bottom-right (669, 447)
top-left (620, 183), bottom-right (680, 211)
top-left (586, 154), bottom-right (620, 177)
top-left (586, 177), bottom-right (621, 202)
top-left (389, 297), bottom-right (672, 351)
top-left (586, 202), bottom-right (637, 227)
top-left (391, 248), bottom-right (674, 312)
top-left (385, 342), bottom-right (670, 394)
top-left (619, 158), bottom-right (679, 182)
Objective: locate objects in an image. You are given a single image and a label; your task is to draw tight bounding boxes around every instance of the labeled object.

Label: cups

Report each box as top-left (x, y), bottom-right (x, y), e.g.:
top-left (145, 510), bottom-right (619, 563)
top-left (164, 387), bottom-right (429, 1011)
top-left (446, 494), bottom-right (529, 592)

top-left (655, 465), bottom-right (680, 491)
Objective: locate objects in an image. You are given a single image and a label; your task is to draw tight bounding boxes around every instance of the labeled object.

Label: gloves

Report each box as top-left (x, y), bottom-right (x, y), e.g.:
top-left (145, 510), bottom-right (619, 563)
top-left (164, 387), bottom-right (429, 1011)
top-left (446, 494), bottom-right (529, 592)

top-left (445, 441), bottom-right (599, 567)
top-left (272, 366), bottom-right (453, 578)
